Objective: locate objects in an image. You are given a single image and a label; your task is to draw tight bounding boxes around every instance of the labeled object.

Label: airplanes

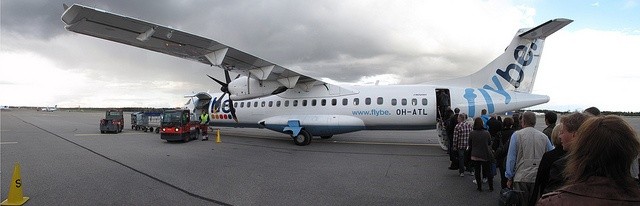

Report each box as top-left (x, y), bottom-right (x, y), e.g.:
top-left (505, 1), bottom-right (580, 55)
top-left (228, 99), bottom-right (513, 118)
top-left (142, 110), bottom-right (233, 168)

top-left (61, 3), bottom-right (573, 150)
top-left (41, 104), bottom-right (57, 112)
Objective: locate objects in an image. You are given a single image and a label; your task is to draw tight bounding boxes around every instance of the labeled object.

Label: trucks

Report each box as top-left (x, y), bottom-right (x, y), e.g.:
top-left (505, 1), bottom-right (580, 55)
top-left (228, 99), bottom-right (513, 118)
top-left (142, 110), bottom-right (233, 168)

top-left (131, 111), bottom-right (162, 134)
top-left (100, 108), bottom-right (124, 134)
top-left (159, 108), bottom-right (201, 142)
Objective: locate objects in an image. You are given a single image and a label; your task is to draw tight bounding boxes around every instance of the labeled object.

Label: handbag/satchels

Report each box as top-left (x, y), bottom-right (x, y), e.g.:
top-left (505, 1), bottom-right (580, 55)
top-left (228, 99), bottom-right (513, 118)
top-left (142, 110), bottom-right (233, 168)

top-left (499, 187), bottom-right (528, 206)
top-left (482, 161), bottom-right (497, 178)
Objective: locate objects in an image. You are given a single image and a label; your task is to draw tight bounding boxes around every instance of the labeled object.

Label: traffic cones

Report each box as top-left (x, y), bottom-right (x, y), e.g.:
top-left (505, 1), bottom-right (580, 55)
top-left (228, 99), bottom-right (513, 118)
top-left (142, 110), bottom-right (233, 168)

top-left (0, 161), bottom-right (30, 206)
top-left (215, 128), bottom-right (221, 143)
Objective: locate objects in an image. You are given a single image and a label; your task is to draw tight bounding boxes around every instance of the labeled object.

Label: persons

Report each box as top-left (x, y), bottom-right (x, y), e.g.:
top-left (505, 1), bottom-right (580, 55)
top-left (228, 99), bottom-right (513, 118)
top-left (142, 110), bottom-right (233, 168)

top-left (542, 112), bottom-right (557, 139)
top-left (198, 110), bottom-right (208, 139)
top-left (505, 112), bottom-right (556, 206)
top-left (438, 90), bottom-right (454, 135)
top-left (448, 108), bottom-right (461, 169)
top-left (453, 114), bottom-right (474, 177)
top-left (536, 115), bottom-right (640, 205)
top-left (545, 112), bottom-right (588, 192)
top-left (511, 113), bottom-right (520, 130)
top-left (496, 118), bottom-right (513, 182)
top-left (585, 107), bottom-right (600, 118)
top-left (472, 118), bottom-right (492, 191)
top-left (463, 112), bottom-right (474, 126)
top-left (480, 110), bottom-right (489, 128)
top-left (486, 114), bottom-right (500, 139)
top-left (533, 123), bottom-right (565, 195)
top-left (497, 116), bottom-right (502, 123)
